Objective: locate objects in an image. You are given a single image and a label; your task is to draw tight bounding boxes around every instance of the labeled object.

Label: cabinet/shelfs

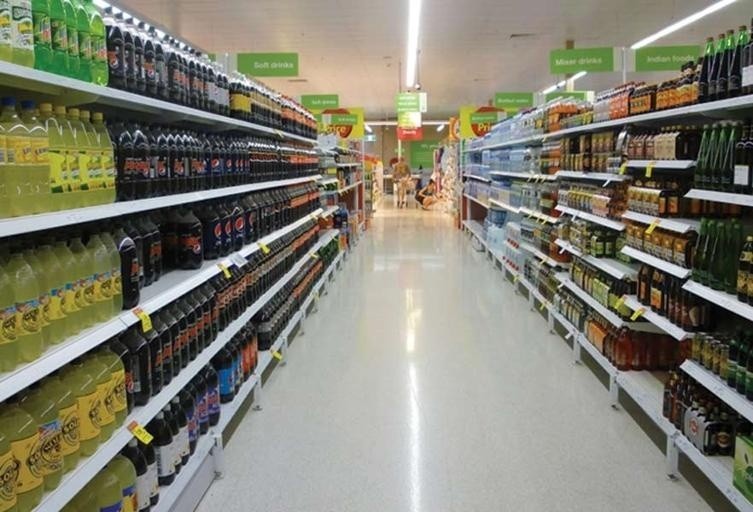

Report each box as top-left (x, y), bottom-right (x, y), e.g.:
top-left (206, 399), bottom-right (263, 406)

top-left (459, 95), bottom-right (753, 512)
top-left (0, 52), bottom-right (361, 512)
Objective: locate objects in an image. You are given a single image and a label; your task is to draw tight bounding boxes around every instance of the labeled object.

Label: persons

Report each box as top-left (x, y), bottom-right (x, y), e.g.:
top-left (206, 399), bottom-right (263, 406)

top-left (391, 156), bottom-right (436, 211)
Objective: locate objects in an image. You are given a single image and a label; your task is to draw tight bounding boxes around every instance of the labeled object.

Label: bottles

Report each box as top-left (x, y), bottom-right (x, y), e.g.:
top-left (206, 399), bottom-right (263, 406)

top-left (661, 23), bottom-right (752, 458)
top-left (1, 0), bottom-right (383, 511)
top-left (460, 51), bottom-right (705, 372)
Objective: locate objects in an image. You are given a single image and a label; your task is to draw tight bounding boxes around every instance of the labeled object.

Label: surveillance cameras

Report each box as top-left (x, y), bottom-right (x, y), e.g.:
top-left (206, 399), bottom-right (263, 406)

top-left (416, 84), bottom-right (421, 90)
